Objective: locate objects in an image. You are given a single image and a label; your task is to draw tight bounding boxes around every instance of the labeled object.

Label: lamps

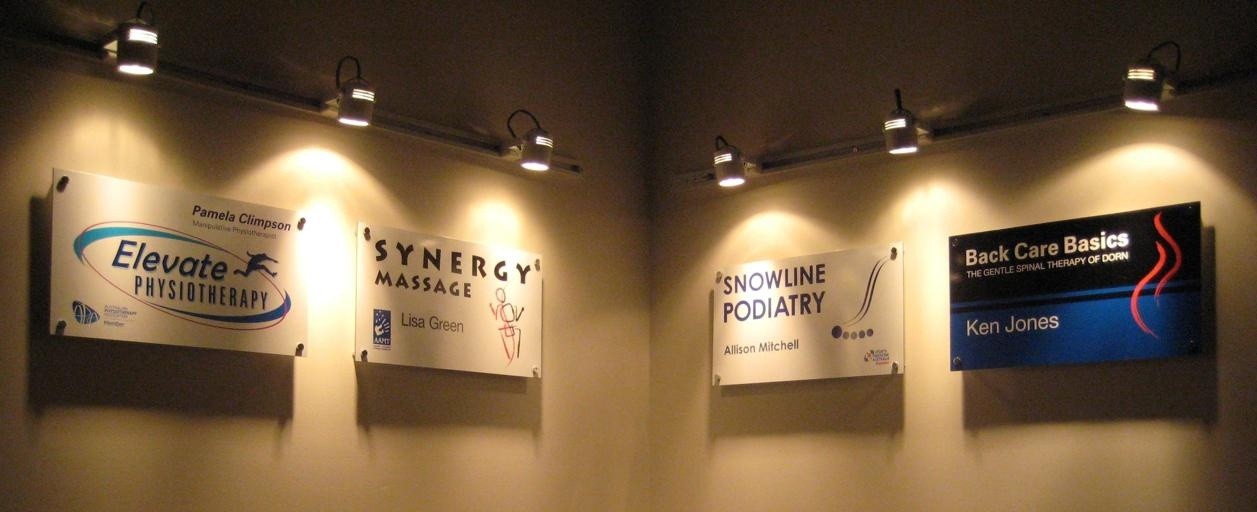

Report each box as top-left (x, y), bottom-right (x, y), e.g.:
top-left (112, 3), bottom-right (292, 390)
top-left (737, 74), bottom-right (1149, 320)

top-left (682, 37), bottom-right (1200, 190)
top-left (14, 2), bottom-right (587, 180)
